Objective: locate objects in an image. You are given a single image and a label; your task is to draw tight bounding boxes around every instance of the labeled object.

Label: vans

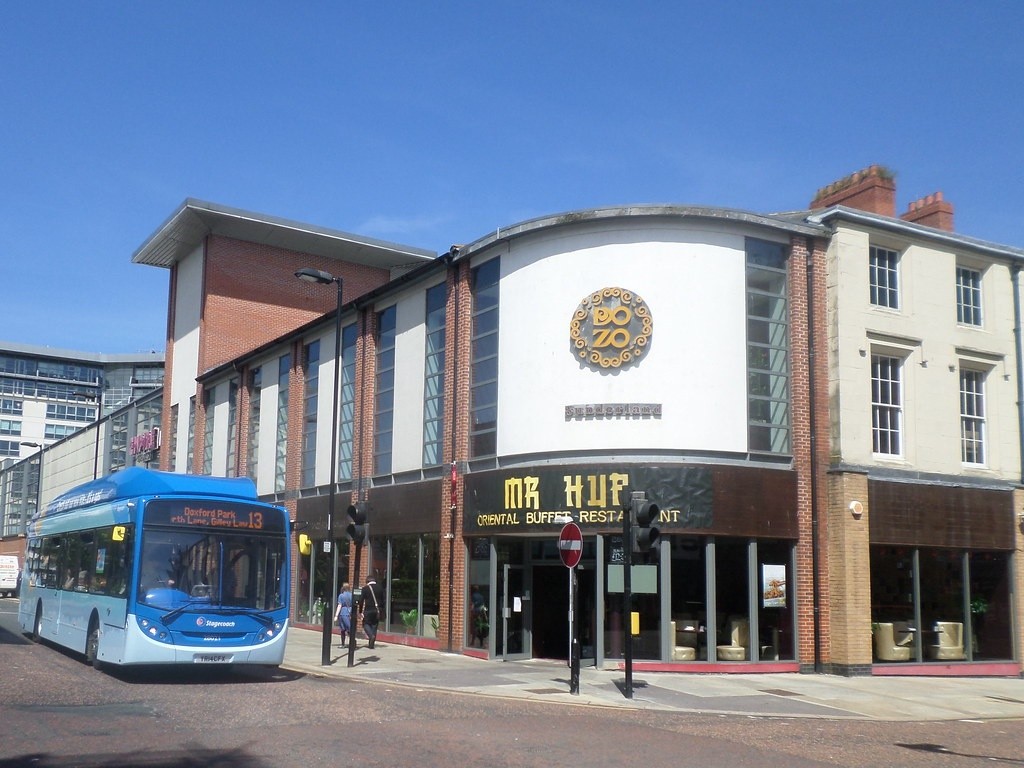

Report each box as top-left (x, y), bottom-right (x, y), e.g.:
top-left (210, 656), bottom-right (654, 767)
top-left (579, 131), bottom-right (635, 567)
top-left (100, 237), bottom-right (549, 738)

top-left (0, 556), bottom-right (20, 597)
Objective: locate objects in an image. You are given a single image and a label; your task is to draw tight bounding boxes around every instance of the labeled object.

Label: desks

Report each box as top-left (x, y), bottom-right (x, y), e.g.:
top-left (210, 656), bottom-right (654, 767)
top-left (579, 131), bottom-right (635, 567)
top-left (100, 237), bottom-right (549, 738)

top-left (676, 629), bottom-right (722, 660)
top-left (898, 630), bottom-right (944, 660)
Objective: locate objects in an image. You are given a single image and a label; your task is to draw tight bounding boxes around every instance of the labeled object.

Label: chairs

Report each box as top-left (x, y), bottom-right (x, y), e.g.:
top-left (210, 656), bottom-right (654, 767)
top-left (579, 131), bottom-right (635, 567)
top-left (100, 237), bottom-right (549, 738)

top-left (929, 621), bottom-right (965, 660)
top-left (718, 620), bottom-right (748, 660)
top-left (658, 620), bottom-right (699, 661)
top-left (874, 622), bottom-right (916, 662)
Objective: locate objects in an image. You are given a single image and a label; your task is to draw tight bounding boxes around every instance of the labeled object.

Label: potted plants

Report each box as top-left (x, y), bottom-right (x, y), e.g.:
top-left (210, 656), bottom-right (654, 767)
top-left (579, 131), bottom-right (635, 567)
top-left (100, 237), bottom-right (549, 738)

top-left (400, 609), bottom-right (418, 635)
top-left (432, 610), bottom-right (440, 637)
top-left (317, 603), bottom-right (324, 625)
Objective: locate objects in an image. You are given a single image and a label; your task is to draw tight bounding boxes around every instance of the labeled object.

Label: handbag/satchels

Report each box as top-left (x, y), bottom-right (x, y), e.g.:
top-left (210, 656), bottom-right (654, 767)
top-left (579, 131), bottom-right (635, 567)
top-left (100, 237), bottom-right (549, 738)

top-left (376, 606), bottom-right (386, 621)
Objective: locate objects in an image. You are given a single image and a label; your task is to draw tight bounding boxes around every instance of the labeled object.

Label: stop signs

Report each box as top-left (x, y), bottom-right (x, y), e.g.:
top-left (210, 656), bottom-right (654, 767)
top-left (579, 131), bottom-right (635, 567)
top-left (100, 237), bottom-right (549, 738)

top-left (559, 523), bottom-right (584, 568)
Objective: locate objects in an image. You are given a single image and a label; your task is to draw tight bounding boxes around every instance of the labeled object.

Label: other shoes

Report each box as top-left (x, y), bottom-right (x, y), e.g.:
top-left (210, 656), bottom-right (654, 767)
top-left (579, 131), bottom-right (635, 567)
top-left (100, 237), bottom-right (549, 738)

top-left (338, 644), bottom-right (346, 648)
top-left (368, 634), bottom-right (376, 649)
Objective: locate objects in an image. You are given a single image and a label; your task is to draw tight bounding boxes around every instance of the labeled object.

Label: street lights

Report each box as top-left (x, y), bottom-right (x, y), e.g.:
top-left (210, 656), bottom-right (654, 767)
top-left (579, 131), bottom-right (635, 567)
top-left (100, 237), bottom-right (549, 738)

top-left (293, 267), bottom-right (344, 665)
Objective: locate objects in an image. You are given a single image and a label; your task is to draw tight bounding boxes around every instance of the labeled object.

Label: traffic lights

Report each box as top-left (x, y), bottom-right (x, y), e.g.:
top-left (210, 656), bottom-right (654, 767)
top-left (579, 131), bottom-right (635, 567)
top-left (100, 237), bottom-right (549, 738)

top-left (346, 500), bottom-right (370, 546)
top-left (631, 501), bottom-right (661, 554)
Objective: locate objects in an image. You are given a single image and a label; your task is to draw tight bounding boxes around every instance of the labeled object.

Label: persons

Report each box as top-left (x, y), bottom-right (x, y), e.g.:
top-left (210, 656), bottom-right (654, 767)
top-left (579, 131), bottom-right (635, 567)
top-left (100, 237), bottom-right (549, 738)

top-left (357, 576), bottom-right (385, 649)
top-left (470, 584), bottom-right (485, 648)
top-left (64, 569), bottom-right (74, 590)
top-left (334, 582), bottom-right (358, 649)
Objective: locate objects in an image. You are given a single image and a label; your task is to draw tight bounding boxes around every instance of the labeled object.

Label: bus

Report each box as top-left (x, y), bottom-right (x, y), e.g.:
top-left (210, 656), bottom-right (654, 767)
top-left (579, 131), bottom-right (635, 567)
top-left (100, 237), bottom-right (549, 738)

top-left (18, 466), bottom-right (312, 670)
